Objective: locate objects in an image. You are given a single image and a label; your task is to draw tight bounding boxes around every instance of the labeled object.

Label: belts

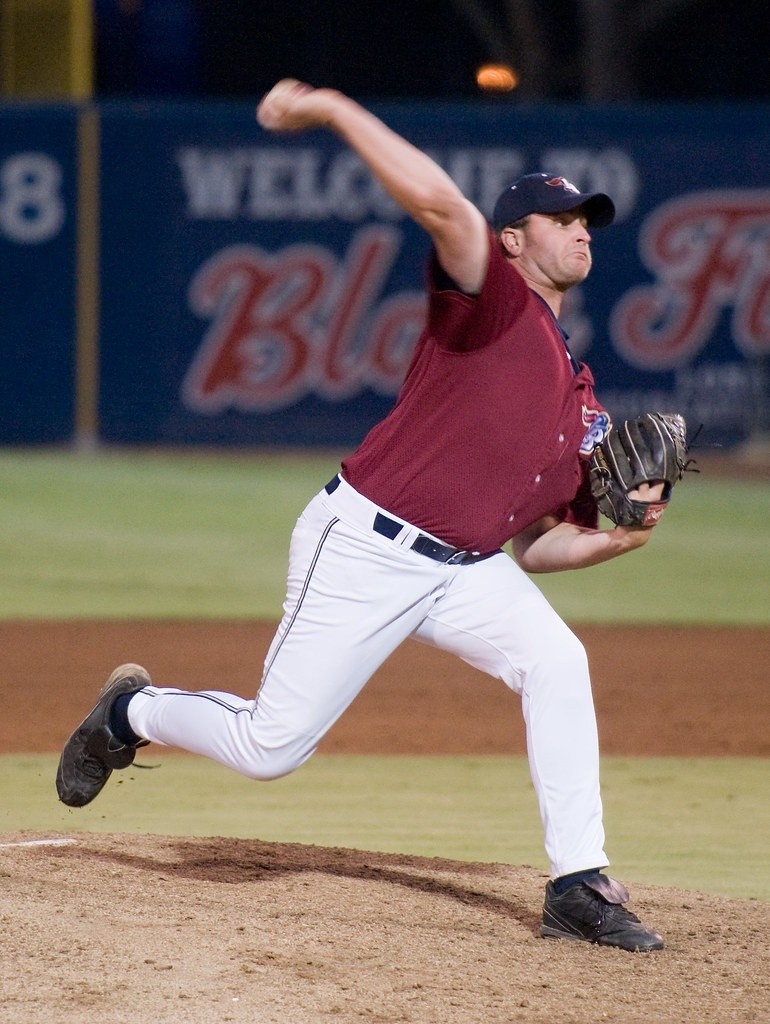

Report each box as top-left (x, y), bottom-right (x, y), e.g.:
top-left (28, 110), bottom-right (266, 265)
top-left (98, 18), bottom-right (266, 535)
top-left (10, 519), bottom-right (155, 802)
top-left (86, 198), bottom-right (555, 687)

top-left (324, 473), bottom-right (505, 565)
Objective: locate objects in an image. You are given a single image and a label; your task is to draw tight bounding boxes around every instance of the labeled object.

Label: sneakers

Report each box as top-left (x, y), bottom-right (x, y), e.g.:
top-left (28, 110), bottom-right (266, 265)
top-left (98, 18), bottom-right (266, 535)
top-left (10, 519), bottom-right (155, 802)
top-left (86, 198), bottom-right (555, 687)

top-left (539, 874), bottom-right (664, 952)
top-left (56, 663), bottom-right (161, 808)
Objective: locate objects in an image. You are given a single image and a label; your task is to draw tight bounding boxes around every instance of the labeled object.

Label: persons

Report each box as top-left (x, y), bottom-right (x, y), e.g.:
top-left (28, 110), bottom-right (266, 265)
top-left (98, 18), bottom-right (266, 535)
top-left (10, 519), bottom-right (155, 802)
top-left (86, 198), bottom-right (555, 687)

top-left (58, 79), bottom-right (665, 951)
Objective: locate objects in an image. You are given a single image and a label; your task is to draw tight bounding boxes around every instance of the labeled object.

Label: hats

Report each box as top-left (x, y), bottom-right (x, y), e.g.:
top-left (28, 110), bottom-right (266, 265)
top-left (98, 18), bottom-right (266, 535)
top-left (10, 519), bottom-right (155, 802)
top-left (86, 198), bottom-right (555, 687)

top-left (493, 173), bottom-right (615, 230)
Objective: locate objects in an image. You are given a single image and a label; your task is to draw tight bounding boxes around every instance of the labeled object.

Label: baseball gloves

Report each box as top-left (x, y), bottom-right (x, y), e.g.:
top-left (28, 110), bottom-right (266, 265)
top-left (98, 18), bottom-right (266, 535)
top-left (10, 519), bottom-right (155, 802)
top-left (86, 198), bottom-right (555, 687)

top-left (588, 412), bottom-right (688, 528)
